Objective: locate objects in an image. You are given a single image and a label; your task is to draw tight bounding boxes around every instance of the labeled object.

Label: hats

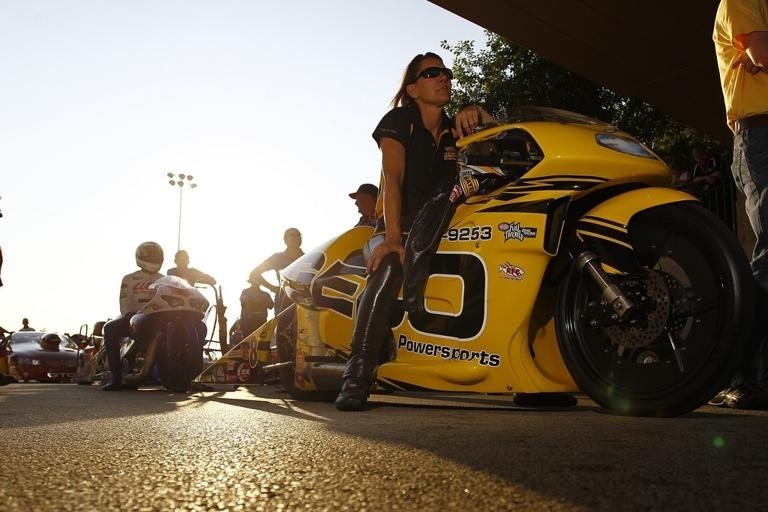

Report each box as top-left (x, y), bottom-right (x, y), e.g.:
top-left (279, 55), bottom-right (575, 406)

top-left (349, 183), bottom-right (379, 199)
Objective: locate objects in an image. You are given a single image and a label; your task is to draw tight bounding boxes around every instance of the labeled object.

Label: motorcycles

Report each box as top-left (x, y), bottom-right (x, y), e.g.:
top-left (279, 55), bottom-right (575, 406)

top-left (120, 275), bottom-right (209, 392)
top-left (275, 103), bottom-right (760, 417)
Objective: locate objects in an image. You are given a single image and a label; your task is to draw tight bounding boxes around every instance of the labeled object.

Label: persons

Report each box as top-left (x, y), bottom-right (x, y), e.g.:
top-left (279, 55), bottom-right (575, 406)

top-left (246, 226), bottom-right (308, 387)
top-left (99, 239), bottom-right (169, 392)
top-left (334, 50), bottom-right (495, 411)
top-left (165, 249), bottom-right (218, 289)
top-left (239, 275), bottom-right (273, 335)
top-left (345, 183), bottom-right (379, 227)
top-left (707, 0), bottom-right (768, 409)
top-left (19, 318), bottom-right (35, 331)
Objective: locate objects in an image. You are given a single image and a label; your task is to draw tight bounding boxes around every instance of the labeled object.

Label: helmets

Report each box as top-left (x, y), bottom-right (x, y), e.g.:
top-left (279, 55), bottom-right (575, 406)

top-left (39, 333), bottom-right (61, 351)
top-left (135, 241), bottom-right (164, 273)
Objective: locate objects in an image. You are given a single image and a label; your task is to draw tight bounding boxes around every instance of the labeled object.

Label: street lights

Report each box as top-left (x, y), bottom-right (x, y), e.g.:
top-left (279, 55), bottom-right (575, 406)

top-left (167, 172), bottom-right (197, 251)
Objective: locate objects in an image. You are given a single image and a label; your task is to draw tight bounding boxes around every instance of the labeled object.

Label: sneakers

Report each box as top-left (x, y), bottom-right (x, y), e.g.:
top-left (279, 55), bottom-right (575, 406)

top-left (102, 380), bottom-right (121, 392)
top-left (335, 376), bottom-right (372, 411)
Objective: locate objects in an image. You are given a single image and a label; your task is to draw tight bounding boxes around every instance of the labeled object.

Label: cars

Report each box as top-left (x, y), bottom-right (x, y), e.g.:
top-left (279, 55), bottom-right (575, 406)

top-left (0, 331), bottom-right (85, 382)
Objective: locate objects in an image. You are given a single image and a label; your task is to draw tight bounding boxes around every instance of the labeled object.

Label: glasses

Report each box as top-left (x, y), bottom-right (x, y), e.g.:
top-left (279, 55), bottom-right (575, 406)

top-left (415, 66), bottom-right (454, 81)
